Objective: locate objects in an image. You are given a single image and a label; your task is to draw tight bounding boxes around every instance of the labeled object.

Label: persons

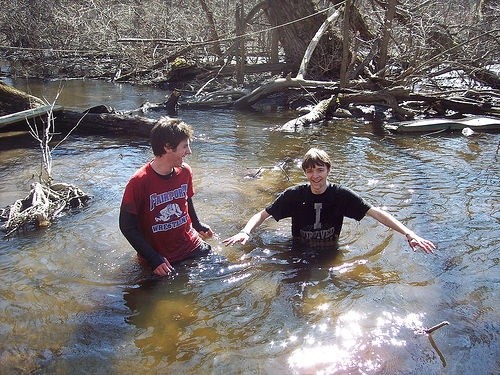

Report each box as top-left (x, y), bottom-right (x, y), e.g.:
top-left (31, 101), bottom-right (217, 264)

top-left (118, 116), bottom-right (213, 276)
top-left (221, 147), bottom-right (436, 255)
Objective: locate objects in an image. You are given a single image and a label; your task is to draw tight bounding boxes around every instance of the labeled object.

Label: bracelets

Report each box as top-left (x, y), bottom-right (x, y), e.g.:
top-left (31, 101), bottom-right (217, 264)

top-left (240, 229), bottom-right (250, 236)
